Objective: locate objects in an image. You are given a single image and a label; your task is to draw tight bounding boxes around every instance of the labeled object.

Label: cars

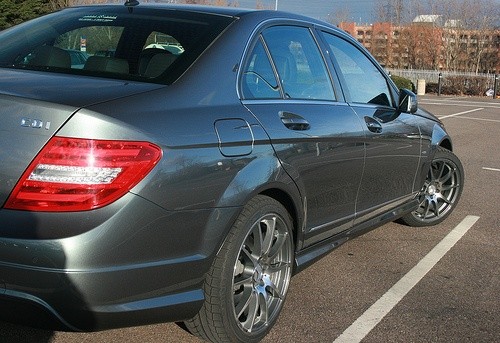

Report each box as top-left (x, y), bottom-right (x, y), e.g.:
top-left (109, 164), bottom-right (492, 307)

top-left (1, 0), bottom-right (465, 343)
top-left (146, 43), bottom-right (183, 53)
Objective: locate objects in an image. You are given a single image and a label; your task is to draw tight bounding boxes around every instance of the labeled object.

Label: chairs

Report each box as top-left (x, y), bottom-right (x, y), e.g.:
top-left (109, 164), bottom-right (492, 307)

top-left (248, 55), bottom-right (292, 98)
top-left (136, 48), bottom-right (175, 77)
top-left (82, 56), bottom-right (129, 75)
top-left (28, 45), bottom-right (72, 69)
